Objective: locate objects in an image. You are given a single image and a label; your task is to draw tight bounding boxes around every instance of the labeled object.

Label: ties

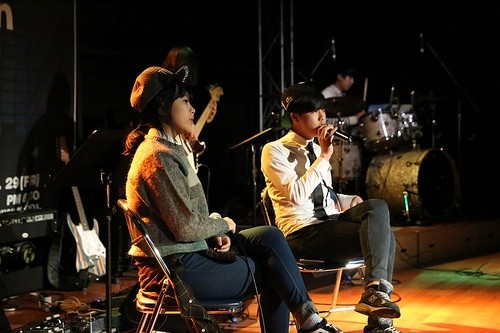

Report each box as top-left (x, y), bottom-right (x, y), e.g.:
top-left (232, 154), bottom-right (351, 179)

top-left (307, 142), bottom-right (330, 220)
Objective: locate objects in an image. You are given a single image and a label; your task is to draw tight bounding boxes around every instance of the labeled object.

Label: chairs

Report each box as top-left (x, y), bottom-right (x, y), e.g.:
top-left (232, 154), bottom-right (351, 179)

top-left (117, 200), bottom-right (245, 333)
top-left (260, 187), bottom-right (366, 313)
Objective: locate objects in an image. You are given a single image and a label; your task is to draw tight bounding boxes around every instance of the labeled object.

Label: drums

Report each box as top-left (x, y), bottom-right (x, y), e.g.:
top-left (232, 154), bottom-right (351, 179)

top-left (329, 106), bottom-right (459, 223)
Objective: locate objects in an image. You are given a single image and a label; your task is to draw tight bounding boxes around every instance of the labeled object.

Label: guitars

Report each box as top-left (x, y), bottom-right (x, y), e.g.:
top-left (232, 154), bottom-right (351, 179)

top-left (175, 85), bottom-right (225, 170)
top-left (58, 135), bottom-right (107, 279)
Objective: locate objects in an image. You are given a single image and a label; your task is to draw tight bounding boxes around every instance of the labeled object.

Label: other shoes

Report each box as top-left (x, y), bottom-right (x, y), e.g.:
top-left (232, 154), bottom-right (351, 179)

top-left (298, 318), bottom-right (343, 333)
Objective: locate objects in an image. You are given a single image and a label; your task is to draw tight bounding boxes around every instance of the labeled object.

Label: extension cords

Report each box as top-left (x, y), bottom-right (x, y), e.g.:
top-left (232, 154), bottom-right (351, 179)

top-left (9, 293), bottom-right (52, 310)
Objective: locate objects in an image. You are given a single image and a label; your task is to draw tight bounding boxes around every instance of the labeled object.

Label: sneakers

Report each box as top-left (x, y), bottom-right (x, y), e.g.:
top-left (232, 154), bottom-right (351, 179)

top-left (354, 278), bottom-right (401, 318)
top-left (364, 318), bottom-right (402, 333)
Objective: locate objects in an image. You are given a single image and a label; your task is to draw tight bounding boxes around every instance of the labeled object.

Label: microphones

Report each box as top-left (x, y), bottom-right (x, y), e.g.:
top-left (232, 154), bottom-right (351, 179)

top-left (332, 39), bottom-right (336, 59)
top-left (420, 33), bottom-right (424, 53)
top-left (209, 212), bottom-right (238, 240)
top-left (327, 129), bottom-right (352, 144)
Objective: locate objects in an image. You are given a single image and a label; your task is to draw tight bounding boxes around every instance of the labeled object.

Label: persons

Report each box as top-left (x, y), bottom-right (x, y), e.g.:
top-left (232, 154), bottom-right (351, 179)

top-left (124, 66), bottom-right (342, 333)
top-left (165, 48), bottom-right (218, 124)
top-left (321, 65), bottom-right (367, 132)
top-left (261, 85), bottom-right (401, 333)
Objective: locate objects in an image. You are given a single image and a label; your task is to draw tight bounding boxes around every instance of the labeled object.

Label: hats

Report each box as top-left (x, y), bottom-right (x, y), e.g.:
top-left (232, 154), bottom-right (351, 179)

top-left (130, 66), bottom-right (189, 112)
